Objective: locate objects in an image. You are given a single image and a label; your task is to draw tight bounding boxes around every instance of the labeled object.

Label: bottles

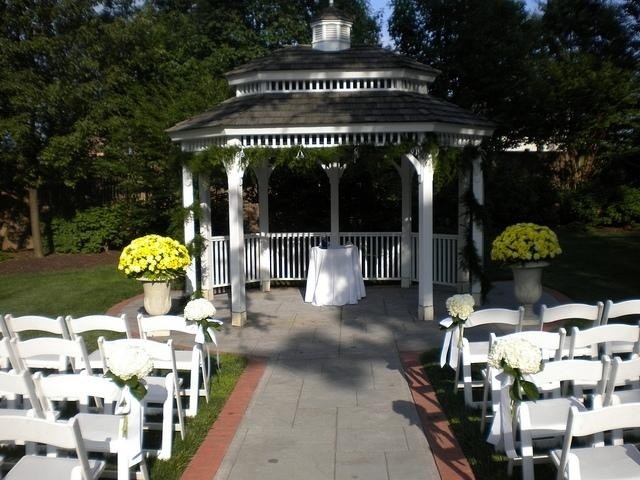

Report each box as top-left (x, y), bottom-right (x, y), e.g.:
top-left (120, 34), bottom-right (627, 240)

top-left (321, 232), bottom-right (327, 249)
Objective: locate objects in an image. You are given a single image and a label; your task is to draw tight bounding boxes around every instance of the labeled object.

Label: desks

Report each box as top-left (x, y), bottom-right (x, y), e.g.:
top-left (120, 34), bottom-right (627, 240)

top-left (304, 244), bottom-right (367, 307)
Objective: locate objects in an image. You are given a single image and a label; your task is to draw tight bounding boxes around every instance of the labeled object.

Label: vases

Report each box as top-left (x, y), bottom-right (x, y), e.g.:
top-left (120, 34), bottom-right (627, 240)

top-left (509, 264), bottom-right (548, 304)
top-left (135, 277), bottom-right (178, 315)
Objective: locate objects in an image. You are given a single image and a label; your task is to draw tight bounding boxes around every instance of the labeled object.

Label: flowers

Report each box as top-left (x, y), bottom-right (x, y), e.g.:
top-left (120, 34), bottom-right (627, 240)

top-left (489, 223), bottom-right (562, 263)
top-left (439, 295), bottom-right (475, 352)
top-left (183, 290), bottom-right (224, 349)
top-left (118, 234), bottom-right (192, 286)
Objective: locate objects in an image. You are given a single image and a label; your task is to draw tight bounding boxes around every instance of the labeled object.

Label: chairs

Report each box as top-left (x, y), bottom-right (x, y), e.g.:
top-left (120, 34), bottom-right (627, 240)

top-left (453, 300), bottom-right (639, 480)
top-left (0, 313), bottom-right (211, 480)
